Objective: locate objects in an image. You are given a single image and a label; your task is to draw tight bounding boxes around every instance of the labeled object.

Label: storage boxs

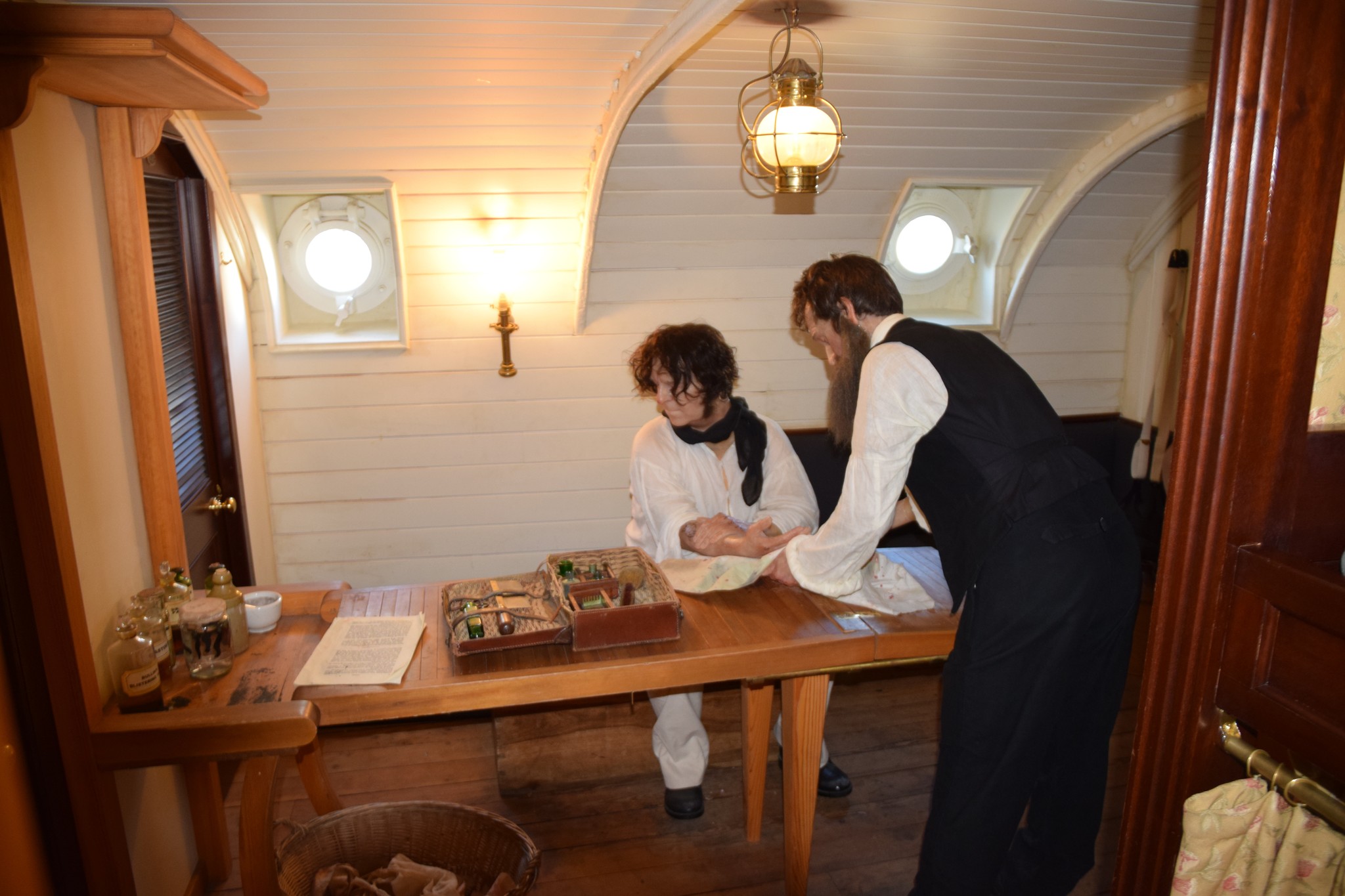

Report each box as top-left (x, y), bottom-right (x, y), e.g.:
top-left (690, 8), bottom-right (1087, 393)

top-left (429, 543), bottom-right (681, 654)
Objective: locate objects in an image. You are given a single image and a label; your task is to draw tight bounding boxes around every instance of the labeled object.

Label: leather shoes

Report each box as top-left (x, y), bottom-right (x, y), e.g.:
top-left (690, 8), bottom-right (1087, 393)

top-left (665, 785), bottom-right (704, 818)
top-left (778, 746), bottom-right (853, 798)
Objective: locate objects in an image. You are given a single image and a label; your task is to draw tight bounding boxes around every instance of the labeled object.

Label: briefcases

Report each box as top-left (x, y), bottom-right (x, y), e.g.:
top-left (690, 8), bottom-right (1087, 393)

top-left (441, 547), bottom-right (684, 657)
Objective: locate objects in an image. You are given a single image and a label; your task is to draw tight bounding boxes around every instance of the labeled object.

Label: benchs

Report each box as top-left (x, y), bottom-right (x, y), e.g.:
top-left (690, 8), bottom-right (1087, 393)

top-left (780, 412), bottom-right (1160, 614)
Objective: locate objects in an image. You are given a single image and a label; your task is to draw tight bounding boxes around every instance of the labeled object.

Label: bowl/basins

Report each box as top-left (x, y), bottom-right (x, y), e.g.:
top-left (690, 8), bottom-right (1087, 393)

top-left (243, 591), bottom-right (282, 633)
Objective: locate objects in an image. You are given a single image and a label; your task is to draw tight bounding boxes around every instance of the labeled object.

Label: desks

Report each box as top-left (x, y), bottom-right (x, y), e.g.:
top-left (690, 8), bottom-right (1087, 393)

top-left (99, 547), bottom-right (959, 896)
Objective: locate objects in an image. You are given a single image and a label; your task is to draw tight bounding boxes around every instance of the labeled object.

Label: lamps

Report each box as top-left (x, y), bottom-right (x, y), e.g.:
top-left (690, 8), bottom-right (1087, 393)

top-left (738, 2), bottom-right (846, 193)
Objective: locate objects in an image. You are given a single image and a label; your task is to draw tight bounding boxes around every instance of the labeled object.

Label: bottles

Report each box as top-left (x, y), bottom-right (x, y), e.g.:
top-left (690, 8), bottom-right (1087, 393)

top-left (107, 561), bottom-right (193, 714)
top-left (179, 596), bottom-right (233, 679)
top-left (202, 563), bottom-right (250, 655)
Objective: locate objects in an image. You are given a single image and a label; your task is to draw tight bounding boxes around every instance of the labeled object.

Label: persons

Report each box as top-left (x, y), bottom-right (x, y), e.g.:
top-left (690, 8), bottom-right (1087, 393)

top-left (759, 254), bottom-right (1140, 896)
top-left (625, 322), bottom-right (855, 821)
